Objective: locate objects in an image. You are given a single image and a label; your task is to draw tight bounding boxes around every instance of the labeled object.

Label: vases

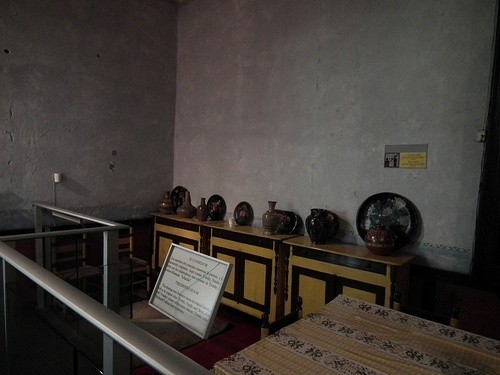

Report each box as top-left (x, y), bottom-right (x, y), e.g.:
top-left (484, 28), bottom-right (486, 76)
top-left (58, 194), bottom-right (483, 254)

top-left (305, 208), bottom-right (332, 245)
top-left (197, 197), bottom-right (209, 221)
top-left (262, 201), bottom-right (278, 235)
top-left (365, 224), bottom-right (395, 256)
top-left (176, 191), bottom-right (197, 218)
top-left (158, 190), bottom-right (175, 215)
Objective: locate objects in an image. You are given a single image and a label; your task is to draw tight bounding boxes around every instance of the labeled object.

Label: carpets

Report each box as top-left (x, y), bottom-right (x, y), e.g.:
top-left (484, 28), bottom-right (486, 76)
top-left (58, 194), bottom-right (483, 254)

top-left (65, 300), bottom-right (230, 371)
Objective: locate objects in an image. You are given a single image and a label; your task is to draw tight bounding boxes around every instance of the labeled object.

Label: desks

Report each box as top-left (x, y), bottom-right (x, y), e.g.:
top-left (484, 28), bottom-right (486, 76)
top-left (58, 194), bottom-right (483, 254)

top-left (214, 295), bottom-right (500, 375)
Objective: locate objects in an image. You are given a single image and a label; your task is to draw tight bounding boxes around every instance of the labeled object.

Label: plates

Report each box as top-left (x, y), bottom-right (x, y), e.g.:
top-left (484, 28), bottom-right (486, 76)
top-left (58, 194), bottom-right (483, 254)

top-left (170, 187), bottom-right (188, 214)
top-left (207, 195), bottom-right (226, 221)
top-left (356, 193), bottom-right (416, 249)
top-left (234, 202), bottom-right (253, 226)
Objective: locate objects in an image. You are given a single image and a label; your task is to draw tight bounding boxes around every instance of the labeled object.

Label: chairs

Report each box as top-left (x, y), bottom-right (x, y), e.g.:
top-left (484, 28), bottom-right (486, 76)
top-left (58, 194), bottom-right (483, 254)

top-left (44, 228), bottom-right (103, 310)
top-left (118, 226), bottom-right (150, 295)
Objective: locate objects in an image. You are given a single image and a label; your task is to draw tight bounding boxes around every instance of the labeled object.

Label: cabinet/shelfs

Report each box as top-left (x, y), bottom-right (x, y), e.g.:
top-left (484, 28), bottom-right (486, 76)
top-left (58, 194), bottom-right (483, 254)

top-left (283, 234), bottom-right (417, 321)
top-left (207, 222), bottom-right (303, 326)
top-left (150, 213), bottom-right (224, 273)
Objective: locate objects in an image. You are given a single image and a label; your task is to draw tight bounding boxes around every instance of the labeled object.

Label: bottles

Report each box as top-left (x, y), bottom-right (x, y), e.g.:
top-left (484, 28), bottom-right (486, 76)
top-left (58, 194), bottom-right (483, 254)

top-left (262, 201), bottom-right (277, 235)
top-left (365, 224), bottom-right (395, 256)
top-left (306, 209), bottom-right (329, 244)
top-left (197, 198), bottom-right (207, 221)
top-left (176, 192), bottom-right (196, 218)
top-left (158, 191), bottom-right (173, 214)
